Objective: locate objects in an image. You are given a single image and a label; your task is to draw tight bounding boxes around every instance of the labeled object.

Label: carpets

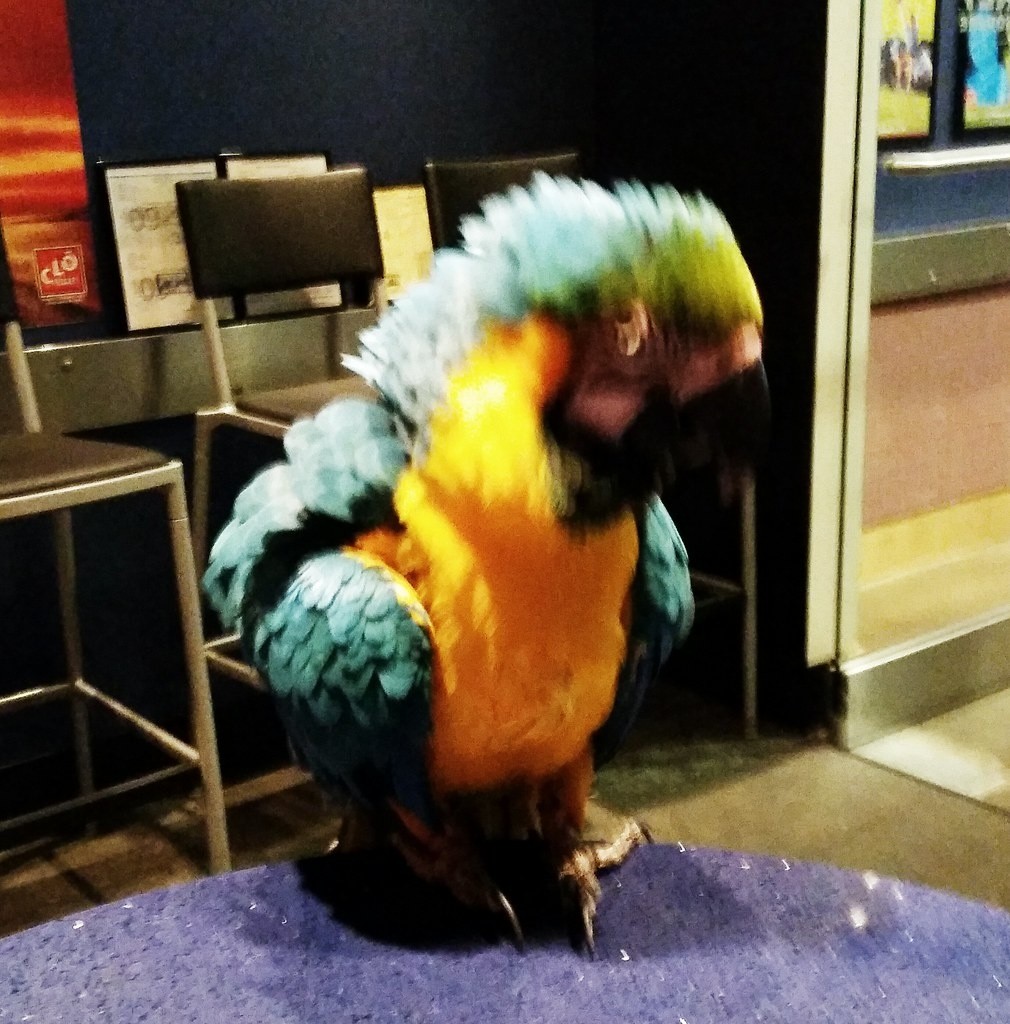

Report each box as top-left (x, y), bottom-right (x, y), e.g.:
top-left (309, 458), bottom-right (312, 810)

top-left (0, 843), bottom-right (1010, 1024)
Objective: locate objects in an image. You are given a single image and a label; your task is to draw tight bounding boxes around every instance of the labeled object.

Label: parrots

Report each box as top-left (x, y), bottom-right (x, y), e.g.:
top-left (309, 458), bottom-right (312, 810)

top-left (203, 168), bottom-right (778, 961)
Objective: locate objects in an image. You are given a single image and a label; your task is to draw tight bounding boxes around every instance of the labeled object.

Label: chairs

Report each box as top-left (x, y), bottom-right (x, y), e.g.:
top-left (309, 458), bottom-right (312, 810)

top-left (174, 162), bottom-right (385, 684)
top-left (422, 152), bottom-right (576, 253)
top-left (0, 230), bottom-right (229, 873)
